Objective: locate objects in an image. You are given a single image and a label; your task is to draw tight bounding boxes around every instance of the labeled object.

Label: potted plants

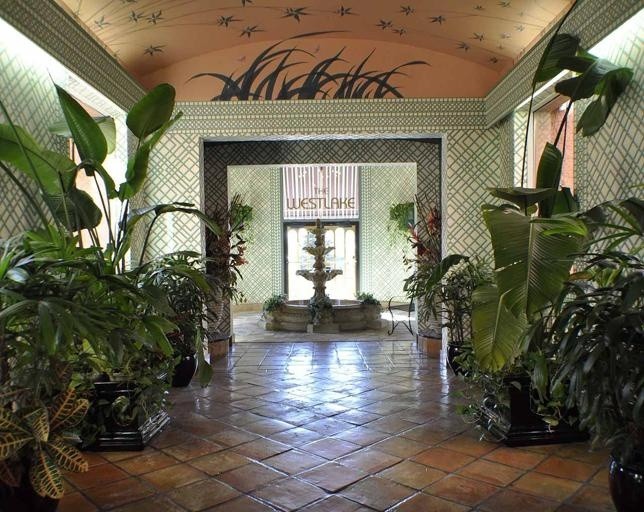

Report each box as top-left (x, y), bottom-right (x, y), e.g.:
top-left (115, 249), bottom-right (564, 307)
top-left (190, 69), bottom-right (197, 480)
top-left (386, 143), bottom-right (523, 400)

top-left (420, 33), bottom-right (644, 510)
top-left (0, 79), bottom-right (250, 512)
